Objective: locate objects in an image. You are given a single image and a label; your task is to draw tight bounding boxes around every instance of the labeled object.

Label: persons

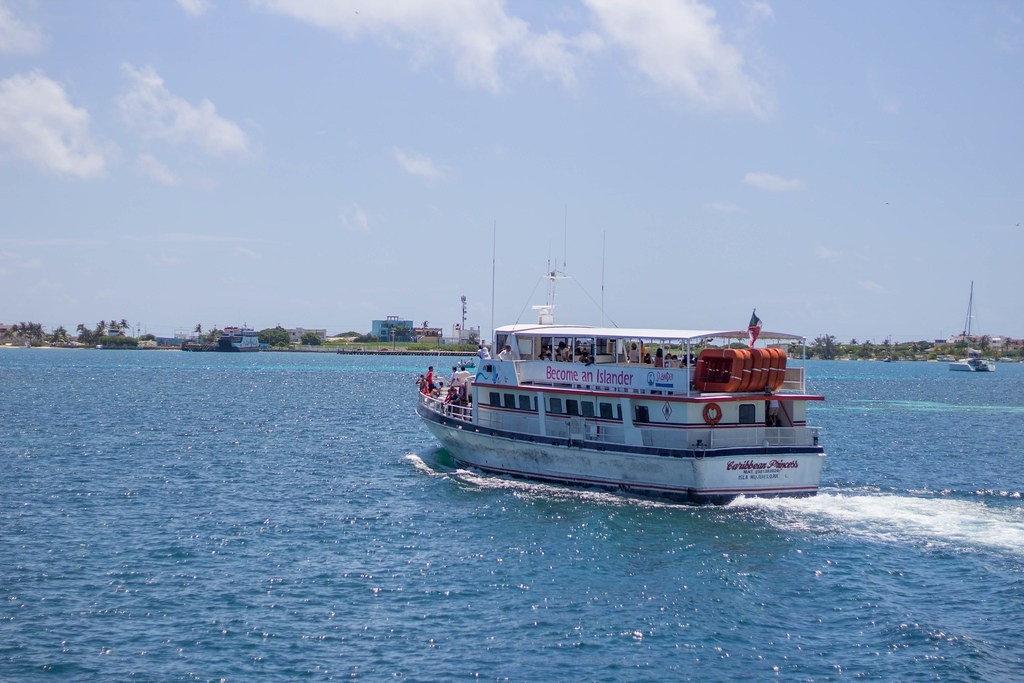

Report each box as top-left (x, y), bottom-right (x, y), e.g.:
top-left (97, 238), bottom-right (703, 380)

top-left (443, 365), bottom-right (471, 418)
top-left (416, 366), bottom-right (443, 399)
top-left (538, 341), bottom-right (594, 366)
top-left (476, 345), bottom-right (491, 360)
top-left (498, 345), bottom-right (512, 361)
top-left (629, 340), bottom-right (698, 368)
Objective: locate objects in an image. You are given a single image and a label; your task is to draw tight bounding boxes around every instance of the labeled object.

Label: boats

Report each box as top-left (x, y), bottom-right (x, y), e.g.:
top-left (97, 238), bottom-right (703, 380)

top-left (217, 322), bottom-right (261, 353)
top-left (413, 203), bottom-right (827, 500)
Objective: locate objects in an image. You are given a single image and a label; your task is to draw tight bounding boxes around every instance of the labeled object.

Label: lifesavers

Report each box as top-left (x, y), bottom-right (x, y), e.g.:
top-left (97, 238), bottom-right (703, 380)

top-left (703, 403), bottom-right (721, 424)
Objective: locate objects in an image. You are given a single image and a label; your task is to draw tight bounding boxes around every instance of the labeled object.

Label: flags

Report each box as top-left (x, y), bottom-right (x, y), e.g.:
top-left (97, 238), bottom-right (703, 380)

top-left (748, 312), bottom-right (762, 348)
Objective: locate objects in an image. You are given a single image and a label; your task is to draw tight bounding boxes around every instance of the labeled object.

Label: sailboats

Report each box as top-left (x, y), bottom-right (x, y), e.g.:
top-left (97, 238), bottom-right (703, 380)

top-left (948, 281), bottom-right (998, 373)
